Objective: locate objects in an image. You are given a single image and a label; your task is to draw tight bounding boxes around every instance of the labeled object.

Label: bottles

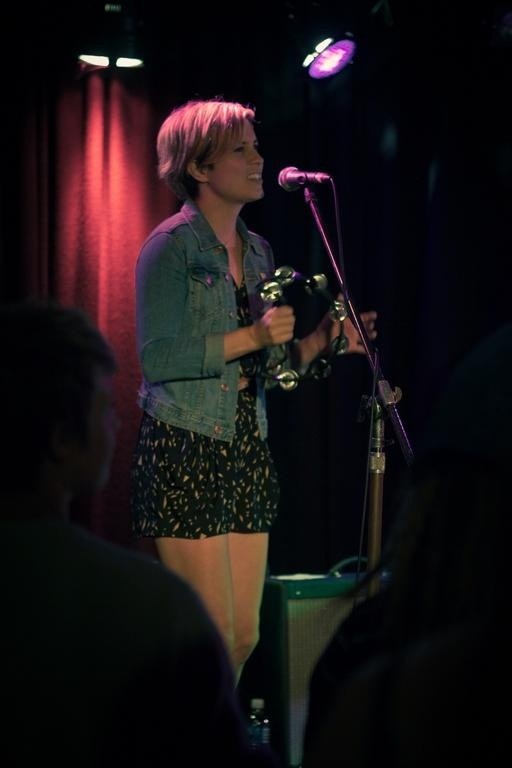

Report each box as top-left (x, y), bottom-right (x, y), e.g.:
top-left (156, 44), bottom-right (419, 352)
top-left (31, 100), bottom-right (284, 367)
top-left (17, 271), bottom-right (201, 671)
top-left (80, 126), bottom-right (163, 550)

top-left (246, 696), bottom-right (272, 752)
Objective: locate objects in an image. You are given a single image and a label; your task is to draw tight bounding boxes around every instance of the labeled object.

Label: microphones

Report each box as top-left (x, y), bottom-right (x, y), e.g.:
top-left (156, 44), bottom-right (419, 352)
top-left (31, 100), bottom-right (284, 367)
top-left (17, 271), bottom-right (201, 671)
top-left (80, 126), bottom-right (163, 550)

top-left (274, 166), bottom-right (331, 194)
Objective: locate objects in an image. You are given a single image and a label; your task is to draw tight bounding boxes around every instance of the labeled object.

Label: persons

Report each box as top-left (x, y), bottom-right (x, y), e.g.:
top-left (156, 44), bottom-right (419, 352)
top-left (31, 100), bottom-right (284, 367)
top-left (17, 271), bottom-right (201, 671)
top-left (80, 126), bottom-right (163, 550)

top-left (0, 298), bottom-right (260, 766)
top-left (133, 98), bottom-right (377, 687)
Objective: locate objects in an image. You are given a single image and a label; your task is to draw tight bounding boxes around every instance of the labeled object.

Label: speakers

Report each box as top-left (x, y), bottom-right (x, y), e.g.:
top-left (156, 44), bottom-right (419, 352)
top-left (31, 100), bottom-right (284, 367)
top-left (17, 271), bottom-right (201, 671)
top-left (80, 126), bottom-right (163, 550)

top-left (258, 572), bottom-right (390, 767)
